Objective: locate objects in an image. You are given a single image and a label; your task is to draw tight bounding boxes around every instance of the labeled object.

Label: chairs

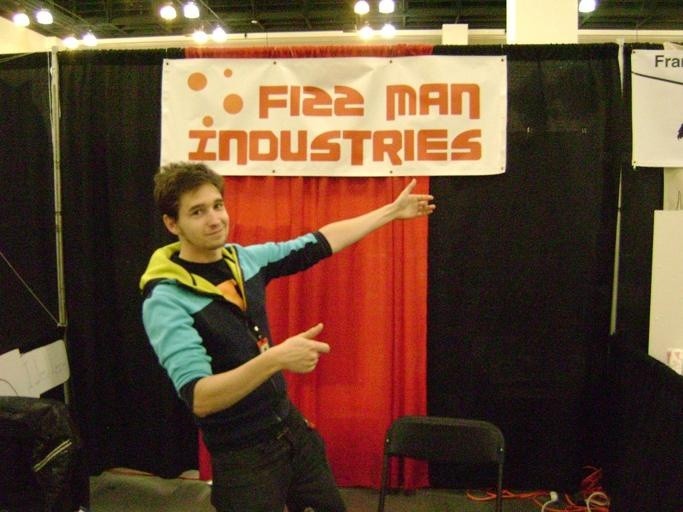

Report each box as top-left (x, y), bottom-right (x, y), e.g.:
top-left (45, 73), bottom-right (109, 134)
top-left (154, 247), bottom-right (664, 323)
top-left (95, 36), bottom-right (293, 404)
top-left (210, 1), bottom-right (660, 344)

top-left (378, 415), bottom-right (506, 512)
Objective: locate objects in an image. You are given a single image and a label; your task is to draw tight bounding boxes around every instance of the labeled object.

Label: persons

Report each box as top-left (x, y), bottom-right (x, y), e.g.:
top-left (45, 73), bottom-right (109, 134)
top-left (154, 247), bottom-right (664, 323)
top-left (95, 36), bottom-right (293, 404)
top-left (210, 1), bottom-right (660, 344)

top-left (137, 160), bottom-right (440, 511)
